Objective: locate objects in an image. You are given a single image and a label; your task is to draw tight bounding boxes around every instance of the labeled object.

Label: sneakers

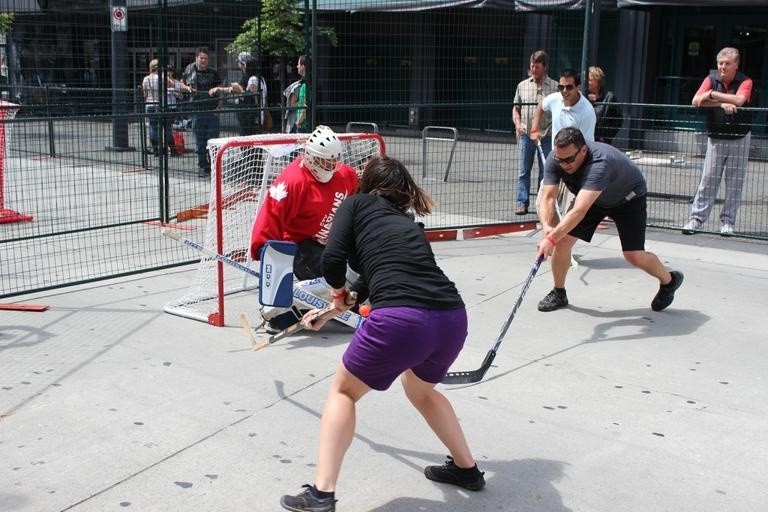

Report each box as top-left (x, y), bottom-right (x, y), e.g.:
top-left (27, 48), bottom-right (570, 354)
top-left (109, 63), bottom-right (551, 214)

top-left (515, 207), bottom-right (528, 215)
top-left (651, 271), bottom-right (683, 311)
top-left (720, 224), bottom-right (734, 237)
top-left (425, 456), bottom-right (485, 491)
top-left (280, 484), bottom-right (338, 512)
top-left (538, 291), bottom-right (568, 311)
top-left (682, 218), bottom-right (702, 234)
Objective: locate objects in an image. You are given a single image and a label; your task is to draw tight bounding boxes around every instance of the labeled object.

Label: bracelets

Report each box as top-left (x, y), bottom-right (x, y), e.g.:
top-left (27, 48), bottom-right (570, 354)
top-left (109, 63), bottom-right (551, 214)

top-left (529, 127), bottom-right (540, 133)
top-left (708, 88), bottom-right (716, 101)
top-left (331, 288), bottom-right (347, 300)
top-left (547, 235), bottom-right (556, 247)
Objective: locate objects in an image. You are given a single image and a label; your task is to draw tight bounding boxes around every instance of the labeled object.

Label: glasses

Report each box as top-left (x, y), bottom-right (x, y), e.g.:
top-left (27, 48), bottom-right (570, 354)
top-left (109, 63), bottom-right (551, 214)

top-left (558, 85), bottom-right (573, 90)
top-left (553, 148), bottom-right (581, 164)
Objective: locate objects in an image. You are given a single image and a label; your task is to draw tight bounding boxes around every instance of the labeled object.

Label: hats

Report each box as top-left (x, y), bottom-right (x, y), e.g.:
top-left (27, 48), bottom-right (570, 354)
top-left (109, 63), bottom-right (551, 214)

top-left (239, 51), bottom-right (252, 64)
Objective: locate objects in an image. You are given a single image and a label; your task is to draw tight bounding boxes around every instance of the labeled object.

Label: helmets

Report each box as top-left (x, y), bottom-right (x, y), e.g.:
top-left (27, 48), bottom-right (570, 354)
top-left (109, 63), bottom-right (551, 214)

top-left (304, 125), bottom-right (343, 184)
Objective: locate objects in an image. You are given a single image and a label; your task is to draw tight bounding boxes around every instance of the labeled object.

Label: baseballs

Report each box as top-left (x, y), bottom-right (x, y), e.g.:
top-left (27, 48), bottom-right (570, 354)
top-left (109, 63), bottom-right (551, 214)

top-left (359, 305), bottom-right (370, 317)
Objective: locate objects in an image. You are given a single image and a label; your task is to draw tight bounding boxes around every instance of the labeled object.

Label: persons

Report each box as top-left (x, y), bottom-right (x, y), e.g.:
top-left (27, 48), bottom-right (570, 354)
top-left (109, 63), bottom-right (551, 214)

top-left (528, 70), bottom-right (597, 256)
top-left (141, 48), bottom-right (270, 177)
top-left (511, 50), bottom-right (560, 216)
top-left (279, 155), bottom-right (486, 512)
top-left (681, 46), bottom-right (755, 236)
top-left (586, 65), bottom-right (624, 145)
top-left (283, 54), bottom-right (314, 134)
top-left (535, 127), bottom-right (683, 313)
top-left (248, 124), bottom-right (427, 334)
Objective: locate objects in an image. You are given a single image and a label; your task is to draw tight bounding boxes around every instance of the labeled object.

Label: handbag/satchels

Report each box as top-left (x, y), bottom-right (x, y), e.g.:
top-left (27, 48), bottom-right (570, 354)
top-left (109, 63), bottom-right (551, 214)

top-left (263, 112), bottom-right (272, 131)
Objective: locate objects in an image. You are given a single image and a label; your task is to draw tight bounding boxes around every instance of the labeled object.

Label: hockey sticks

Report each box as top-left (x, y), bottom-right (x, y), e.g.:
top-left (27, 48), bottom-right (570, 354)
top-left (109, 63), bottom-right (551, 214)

top-left (537, 139), bottom-right (578, 273)
top-left (161, 227), bottom-right (365, 351)
top-left (440, 253), bottom-right (544, 383)
